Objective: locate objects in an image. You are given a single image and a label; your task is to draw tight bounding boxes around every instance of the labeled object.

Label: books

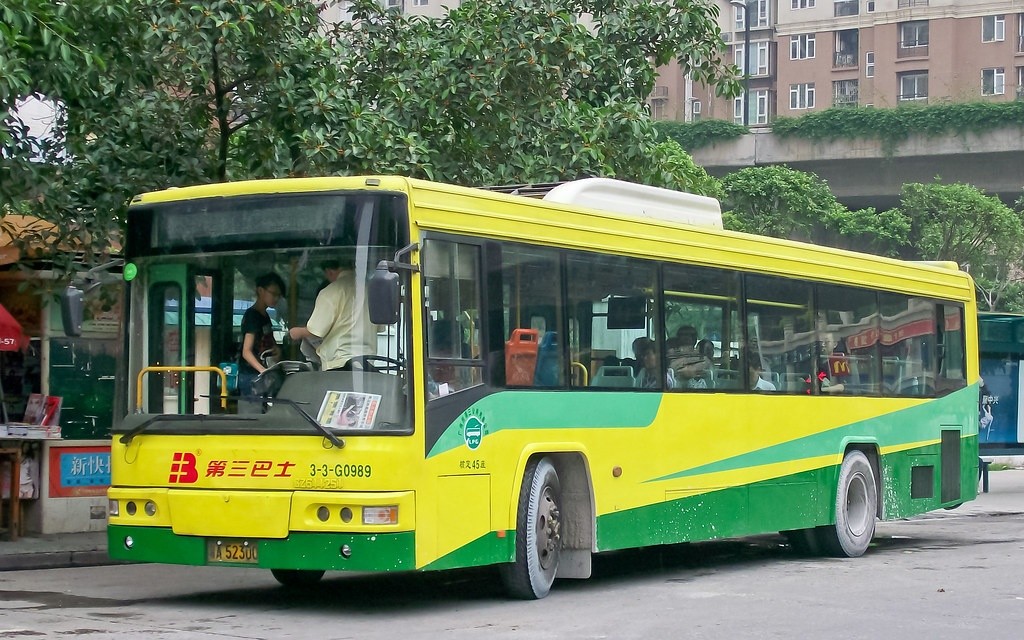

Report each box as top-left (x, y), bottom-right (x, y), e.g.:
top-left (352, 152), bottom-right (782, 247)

top-left (0, 393), bottom-right (66, 499)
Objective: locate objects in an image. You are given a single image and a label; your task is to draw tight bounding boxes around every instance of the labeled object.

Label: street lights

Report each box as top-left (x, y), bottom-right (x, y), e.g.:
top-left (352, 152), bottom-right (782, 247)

top-left (728, 1), bottom-right (754, 133)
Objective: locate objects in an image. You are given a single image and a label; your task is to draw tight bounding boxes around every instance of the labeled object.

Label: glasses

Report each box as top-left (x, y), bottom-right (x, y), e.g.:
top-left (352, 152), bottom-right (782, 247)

top-left (263, 288), bottom-right (283, 299)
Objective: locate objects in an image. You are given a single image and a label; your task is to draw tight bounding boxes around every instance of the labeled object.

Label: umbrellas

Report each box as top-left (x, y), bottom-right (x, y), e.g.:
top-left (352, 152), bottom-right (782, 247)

top-left (0, 304), bottom-right (31, 422)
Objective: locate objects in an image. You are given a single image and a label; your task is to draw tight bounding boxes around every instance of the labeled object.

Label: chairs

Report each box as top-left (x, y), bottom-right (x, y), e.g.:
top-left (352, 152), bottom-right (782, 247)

top-left (505, 329), bottom-right (540, 386)
top-left (590, 352), bottom-right (930, 397)
top-left (532, 332), bottom-right (559, 385)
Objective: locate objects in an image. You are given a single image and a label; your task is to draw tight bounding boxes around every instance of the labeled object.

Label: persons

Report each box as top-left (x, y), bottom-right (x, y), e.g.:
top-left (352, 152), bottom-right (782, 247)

top-left (16, 441), bottom-right (36, 498)
top-left (235, 251), bottom-right (386, 415)
top-left (338, 405), bottom-right (357, 426)
top-left (632, 324), bottom-right (852, 394)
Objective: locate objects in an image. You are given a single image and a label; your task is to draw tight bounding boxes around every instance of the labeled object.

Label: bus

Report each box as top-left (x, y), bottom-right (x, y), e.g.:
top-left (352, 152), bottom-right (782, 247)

top-left (59, 175), bottom-right (981, 601)
